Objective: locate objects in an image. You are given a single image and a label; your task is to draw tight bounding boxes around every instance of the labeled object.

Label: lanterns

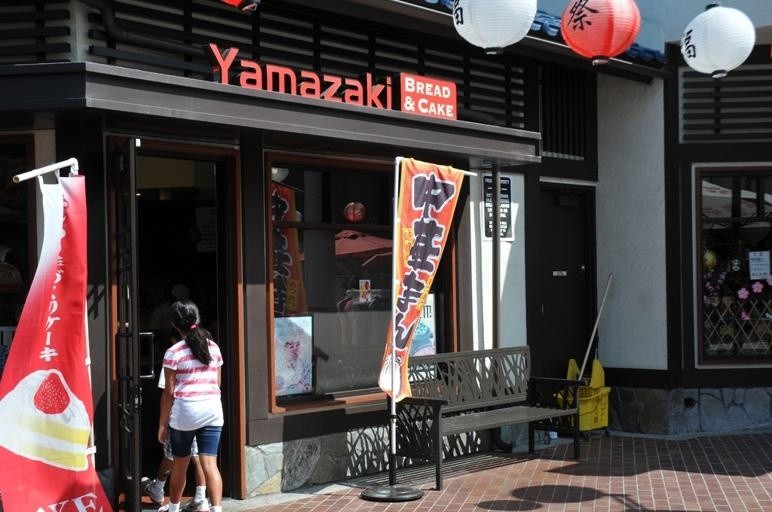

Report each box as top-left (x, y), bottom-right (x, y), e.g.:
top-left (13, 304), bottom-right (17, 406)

top-left (561, 1), bottom-right (640, 69)
top-left (450, 1), bottom-right (539, 60)
top-left (680, 3), bottom-right (758, 85)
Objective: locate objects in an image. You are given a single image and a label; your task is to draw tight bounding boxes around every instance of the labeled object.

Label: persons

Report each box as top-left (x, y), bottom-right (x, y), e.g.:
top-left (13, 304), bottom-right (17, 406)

top-left (155, 300), bottom-right (231, 511)
top-left (272, 340), bottom-right (311, 394)
top-left (140, 325), bottom-right (209, 508)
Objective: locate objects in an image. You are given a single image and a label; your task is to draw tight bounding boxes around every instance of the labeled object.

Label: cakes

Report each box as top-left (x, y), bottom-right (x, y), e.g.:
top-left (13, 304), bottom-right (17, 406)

top-left (0, 368), bottom-right (90, 472)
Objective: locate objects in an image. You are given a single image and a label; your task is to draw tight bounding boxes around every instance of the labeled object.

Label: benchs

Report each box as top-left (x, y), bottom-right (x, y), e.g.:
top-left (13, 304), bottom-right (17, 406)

top-left (387, 345), bottom-right (588, 490)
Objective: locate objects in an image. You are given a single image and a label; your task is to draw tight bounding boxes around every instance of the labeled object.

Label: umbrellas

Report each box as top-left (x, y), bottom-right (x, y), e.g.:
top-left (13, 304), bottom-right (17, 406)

top-left (300, 230), bottom-right (398, 290)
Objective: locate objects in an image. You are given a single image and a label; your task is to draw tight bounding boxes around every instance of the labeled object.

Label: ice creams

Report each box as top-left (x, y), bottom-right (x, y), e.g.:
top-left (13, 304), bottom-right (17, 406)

top-left (284, 340), bottom-right (300, 370)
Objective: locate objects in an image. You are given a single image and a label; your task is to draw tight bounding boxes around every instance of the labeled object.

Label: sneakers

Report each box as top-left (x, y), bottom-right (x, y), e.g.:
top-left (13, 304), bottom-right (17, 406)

top-left (141, 474), bottom-right (166, 507)
top-left (157, 503), bottom-right (169, 512)
top-left (181, 497), bottom-right (210, 511)
